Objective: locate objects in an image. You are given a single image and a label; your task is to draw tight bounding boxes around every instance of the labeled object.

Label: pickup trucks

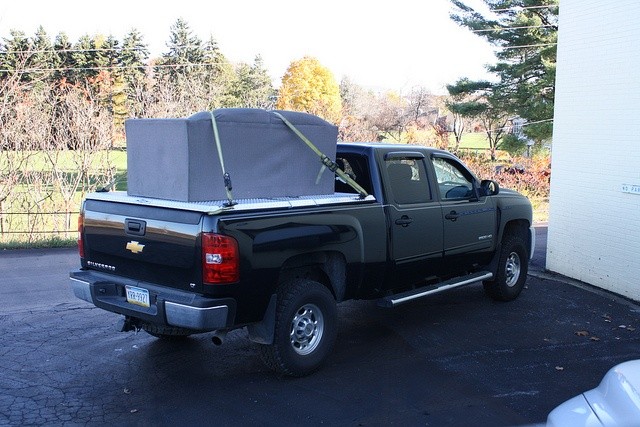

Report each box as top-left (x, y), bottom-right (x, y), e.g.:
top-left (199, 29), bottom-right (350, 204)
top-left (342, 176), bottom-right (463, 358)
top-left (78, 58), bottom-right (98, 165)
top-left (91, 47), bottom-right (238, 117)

top-left (69, 144), bottom-right (535, 376)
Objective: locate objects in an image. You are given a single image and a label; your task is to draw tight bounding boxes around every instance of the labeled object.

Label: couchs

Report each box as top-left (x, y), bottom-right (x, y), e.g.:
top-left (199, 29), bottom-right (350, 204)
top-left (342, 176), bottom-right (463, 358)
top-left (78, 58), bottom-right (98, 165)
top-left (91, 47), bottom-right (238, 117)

top-left (124, 107), bottom-right (338, 203)
